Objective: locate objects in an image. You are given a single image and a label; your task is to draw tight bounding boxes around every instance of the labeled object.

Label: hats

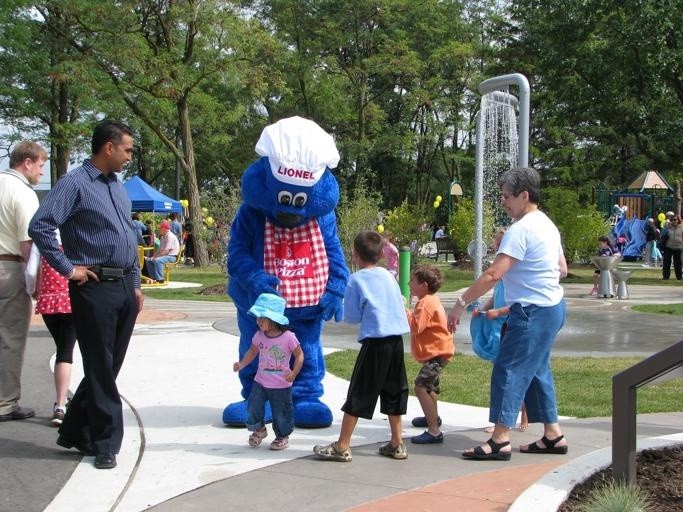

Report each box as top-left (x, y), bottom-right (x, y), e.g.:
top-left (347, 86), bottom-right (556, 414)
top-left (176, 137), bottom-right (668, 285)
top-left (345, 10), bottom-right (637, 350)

top-left (157, 222), bottom-right (171, 230)
top-left (247, 291), bottom-right (290, 327)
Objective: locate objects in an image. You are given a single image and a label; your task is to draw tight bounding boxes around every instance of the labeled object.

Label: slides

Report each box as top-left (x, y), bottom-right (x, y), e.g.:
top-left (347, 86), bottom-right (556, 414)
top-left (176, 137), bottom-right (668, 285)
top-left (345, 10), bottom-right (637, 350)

top-left (601, 212), bottom-right (649, 258)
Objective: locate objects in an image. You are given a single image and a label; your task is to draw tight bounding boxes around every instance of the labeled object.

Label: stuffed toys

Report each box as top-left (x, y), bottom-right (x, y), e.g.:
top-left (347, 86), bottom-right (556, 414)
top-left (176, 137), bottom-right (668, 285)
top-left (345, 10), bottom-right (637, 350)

top-left (222, 116), bottom-right (349, 428)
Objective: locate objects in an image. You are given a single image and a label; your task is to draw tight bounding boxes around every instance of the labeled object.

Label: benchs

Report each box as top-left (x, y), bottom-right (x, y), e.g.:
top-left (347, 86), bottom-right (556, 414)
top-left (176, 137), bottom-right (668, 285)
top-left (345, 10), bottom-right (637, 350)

top-left (434, 237), bottom-right (462, 262)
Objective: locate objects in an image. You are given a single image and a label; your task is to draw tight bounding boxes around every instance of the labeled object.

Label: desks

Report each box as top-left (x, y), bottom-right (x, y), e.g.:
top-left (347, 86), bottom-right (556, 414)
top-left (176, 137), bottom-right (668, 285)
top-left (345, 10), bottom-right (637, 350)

top-left (136, 245), bottom-right (156, 284)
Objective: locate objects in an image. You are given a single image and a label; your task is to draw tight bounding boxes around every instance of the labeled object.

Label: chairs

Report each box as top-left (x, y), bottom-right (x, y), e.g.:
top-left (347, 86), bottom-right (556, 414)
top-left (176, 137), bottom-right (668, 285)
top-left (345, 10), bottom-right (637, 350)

top-left (158, 244), bottom-right (186, 286)
top-left (137, 246), bottom-right (144, 270)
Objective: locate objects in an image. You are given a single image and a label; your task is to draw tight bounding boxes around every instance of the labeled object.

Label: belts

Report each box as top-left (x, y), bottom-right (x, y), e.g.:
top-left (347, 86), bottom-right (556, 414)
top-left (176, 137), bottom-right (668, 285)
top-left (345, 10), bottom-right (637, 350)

top-left (0, 254), bottom-right (25, 262)
top-left (96, 268), bottom-right (132, 281)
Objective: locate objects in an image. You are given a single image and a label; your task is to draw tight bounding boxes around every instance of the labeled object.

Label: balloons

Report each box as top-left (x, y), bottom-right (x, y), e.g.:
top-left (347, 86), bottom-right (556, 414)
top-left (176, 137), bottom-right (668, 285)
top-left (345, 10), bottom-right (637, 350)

top-left (434, 200), bottom-right (440, 209)
top-left (436, 195), bottom-right (443, 202)
top-left (179, 199), bottom-right (189, 209)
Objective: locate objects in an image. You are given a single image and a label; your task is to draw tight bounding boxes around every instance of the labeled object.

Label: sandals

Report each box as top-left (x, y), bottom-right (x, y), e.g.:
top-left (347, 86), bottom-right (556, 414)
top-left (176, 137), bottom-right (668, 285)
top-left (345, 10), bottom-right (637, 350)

top-left (411, 432), bottom-right (444, 443)
top-left (519, 434), bottom-right (569, 454)
top-left (412, 417), bottom-right (442, 428)
top-left (461, 438), bottom-right (513, 461)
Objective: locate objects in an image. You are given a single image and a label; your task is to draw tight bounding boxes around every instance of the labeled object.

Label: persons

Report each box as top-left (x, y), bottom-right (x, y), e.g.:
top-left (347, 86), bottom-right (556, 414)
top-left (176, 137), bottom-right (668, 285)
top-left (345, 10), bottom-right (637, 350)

top-left (0, 142), bottom-right (47, 425)
top-left (446, 168), bottom-right (568, 460)
top-left (25, 228), bottom-right (74, 426)
top-left (589, 209), bottom-right (682, 296)
top-left (234, 293), bottom-right (304, 450)
top-left (27, 119), bottom-right (144, 470)
top-left (128, 212), bottom-right (223, 284)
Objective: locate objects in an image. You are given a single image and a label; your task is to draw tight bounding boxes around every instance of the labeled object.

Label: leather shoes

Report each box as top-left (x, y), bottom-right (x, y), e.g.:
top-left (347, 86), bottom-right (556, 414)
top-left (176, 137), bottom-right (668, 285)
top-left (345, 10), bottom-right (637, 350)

top-left (0, 406), bottom-right (35, 422)
top-left (56, 433), bottom-right (96, 456)
top-left (94, 451), bottom-right (117, 469)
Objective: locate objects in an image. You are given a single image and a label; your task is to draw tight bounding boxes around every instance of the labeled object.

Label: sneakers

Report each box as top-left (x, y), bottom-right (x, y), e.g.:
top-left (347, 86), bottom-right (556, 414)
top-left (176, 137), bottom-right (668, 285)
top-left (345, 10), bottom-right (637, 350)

top-left (378, 442), bottom-right (407, 459)
top-left (248, 427), bottom-right (268, 447)
top-left (313, 442), bottom-right (352, 462)
top-left (269, 435), bottom-right (288, 450)
top-left (50, 397), bottom-right (74, 425)
